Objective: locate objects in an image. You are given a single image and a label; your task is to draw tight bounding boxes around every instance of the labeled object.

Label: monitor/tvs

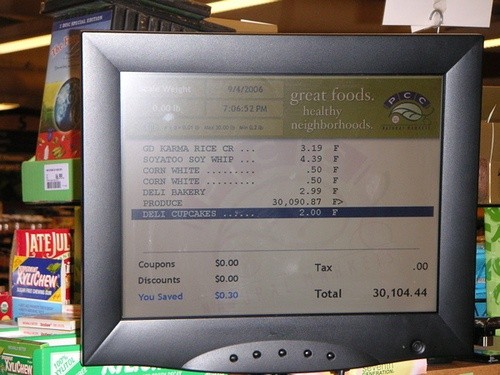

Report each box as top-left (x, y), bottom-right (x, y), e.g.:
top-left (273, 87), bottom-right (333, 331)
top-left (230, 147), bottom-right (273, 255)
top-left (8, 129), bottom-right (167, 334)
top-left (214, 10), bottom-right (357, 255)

top-left (80, 29), bottom-right (484, 371)
